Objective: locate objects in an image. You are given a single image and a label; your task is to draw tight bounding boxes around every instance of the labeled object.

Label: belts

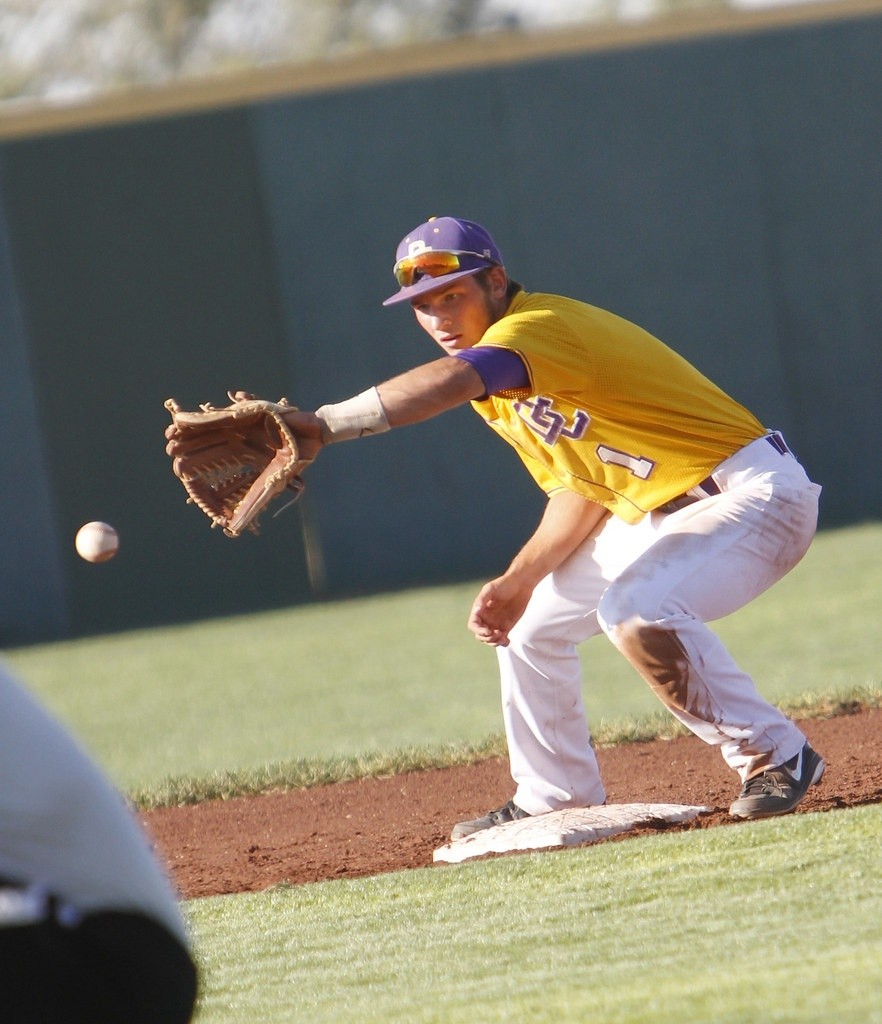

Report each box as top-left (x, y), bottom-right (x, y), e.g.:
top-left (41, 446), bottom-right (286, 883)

top-left (1, 884), bottom-right (201, 1000)
top-left (654, 433), bottom-right (789, 514)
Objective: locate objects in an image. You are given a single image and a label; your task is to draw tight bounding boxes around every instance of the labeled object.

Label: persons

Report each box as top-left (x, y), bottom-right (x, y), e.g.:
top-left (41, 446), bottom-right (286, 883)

top-left (0, 664), bottom-right (201, 1024)
top-left (166, 213), bottom-right (827, 842)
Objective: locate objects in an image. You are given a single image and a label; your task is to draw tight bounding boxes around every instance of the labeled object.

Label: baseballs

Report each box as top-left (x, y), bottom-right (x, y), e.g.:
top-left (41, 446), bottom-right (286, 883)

top-left (74, 521), bottom-right (118, 563)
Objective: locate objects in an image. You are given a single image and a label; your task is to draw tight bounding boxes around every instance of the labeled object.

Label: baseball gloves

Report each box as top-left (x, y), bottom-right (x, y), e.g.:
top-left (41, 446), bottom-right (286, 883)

top-left (162, 391), bottom-right (312, 540)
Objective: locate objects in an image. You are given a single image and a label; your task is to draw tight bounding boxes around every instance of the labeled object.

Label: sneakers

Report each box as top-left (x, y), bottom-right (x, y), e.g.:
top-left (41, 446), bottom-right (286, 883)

top-left (730, 741), bottom-right (825, 820)
top-left (451, 798), bottom-right (530, 842)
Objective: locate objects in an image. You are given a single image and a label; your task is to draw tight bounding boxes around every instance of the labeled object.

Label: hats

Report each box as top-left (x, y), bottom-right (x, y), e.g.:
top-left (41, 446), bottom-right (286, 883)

top-left (380, 215), bottom-right (506, 307)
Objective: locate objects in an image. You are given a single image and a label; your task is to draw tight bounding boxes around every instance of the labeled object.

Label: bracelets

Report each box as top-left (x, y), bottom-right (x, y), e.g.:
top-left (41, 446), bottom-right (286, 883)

top-left (315, 384), bottom-right (390, 447)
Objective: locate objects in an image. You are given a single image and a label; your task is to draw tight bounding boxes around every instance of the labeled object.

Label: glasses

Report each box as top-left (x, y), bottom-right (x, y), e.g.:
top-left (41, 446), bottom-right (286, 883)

top-left (393, 251), bottom-right (505, 288)
